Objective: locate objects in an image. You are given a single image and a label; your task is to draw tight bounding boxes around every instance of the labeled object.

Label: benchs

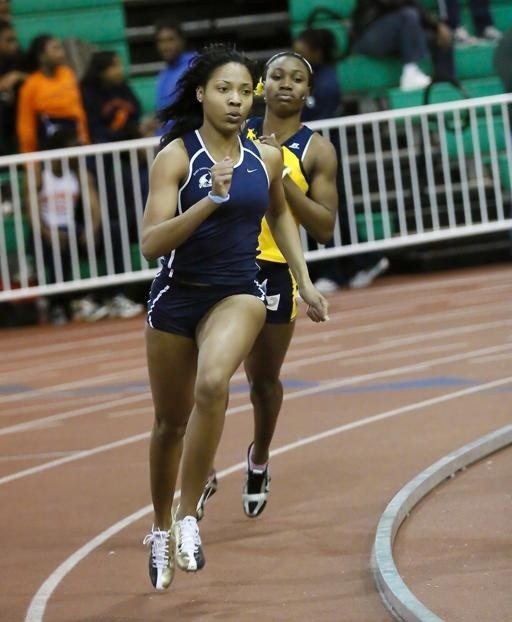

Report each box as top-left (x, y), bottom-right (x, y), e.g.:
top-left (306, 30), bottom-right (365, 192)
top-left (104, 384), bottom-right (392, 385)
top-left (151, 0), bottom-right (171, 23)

top-left (0, 0), bottom-right (396, 291)
top-left (289, 0), bottom-right (510, 187)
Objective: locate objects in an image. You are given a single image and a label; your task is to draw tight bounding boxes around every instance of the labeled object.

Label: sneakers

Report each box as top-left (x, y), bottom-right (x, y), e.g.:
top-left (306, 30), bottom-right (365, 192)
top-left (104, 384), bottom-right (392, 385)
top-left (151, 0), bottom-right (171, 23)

top-left (400, 61), bottom-right (433, 92)
top-left (108, 293), bottom-right (145, 321)
top-left (70, 298), bottom-right (110, 324)
top-left (172, 469), bottom-right (219, 522)
top-left (142, 521), bottom-right (176, 591)
top-left (347, 257), bottom-right (390, 290)
top-left (172, 503), bottom-right (206, 574)
top-left (295, 277), bottom-right (341, 304)
top-left (241, 440), bottom-right (271, 519)
top-left (452, 26), bottom-right (481, 49)
top-left (482, 24), bottom-right (507, 43)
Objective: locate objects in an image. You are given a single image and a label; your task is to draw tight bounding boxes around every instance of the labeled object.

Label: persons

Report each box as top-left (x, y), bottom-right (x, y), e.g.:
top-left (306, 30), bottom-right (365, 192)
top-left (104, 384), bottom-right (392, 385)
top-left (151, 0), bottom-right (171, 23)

top-left (196, 51), bottom-right (337, 521)
top-left (139, 43), bottom-right (330, 592)
